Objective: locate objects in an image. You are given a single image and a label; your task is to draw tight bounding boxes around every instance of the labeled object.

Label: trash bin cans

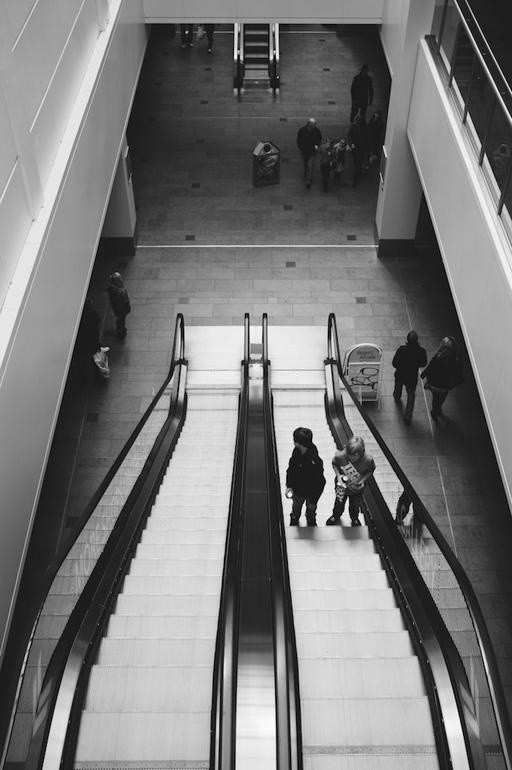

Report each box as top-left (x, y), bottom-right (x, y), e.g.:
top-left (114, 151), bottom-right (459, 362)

top-left (253, 142), bottom-right (280, 187)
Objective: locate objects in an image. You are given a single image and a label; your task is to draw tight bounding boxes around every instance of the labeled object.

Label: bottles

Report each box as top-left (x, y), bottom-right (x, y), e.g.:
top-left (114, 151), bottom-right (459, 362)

top-left (342, 476), bottom-right (350, 484)
top-left (287, 491), bottom-right (294, 499)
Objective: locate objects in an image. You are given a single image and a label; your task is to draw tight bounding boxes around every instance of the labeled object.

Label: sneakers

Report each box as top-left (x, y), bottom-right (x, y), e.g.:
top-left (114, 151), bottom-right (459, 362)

top-left (326, 515), bottom-right (340, 524)
top-left (308, 521), bottom-right (317, 527)
top-left (291, 520), bottom-right (299, 526)
top-left (353, 519), bottom-right (361, 526)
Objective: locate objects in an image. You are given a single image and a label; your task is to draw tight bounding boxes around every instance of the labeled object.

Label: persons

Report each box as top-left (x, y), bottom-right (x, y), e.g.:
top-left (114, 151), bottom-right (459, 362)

top-left (294, 107), bottom-right (385, 197)
top-left (199, 24), bottom-right (219, 54)
top-left (348, 63), bottom-right (376, 125)
top-left (324, 434), bottom-right (377, 528)
top-left (418, 335), bottom-right (458, 420)
top-left (91, 342), bottom-right (115, 386)
top-left (391, 329), bottom-right (423, 425)
top-left (106, 270), bottom-right (134, 341)
top-left (178, 24), bottom-right (195, 49)
top-left (284, 425), bottom-right (327, 528)
